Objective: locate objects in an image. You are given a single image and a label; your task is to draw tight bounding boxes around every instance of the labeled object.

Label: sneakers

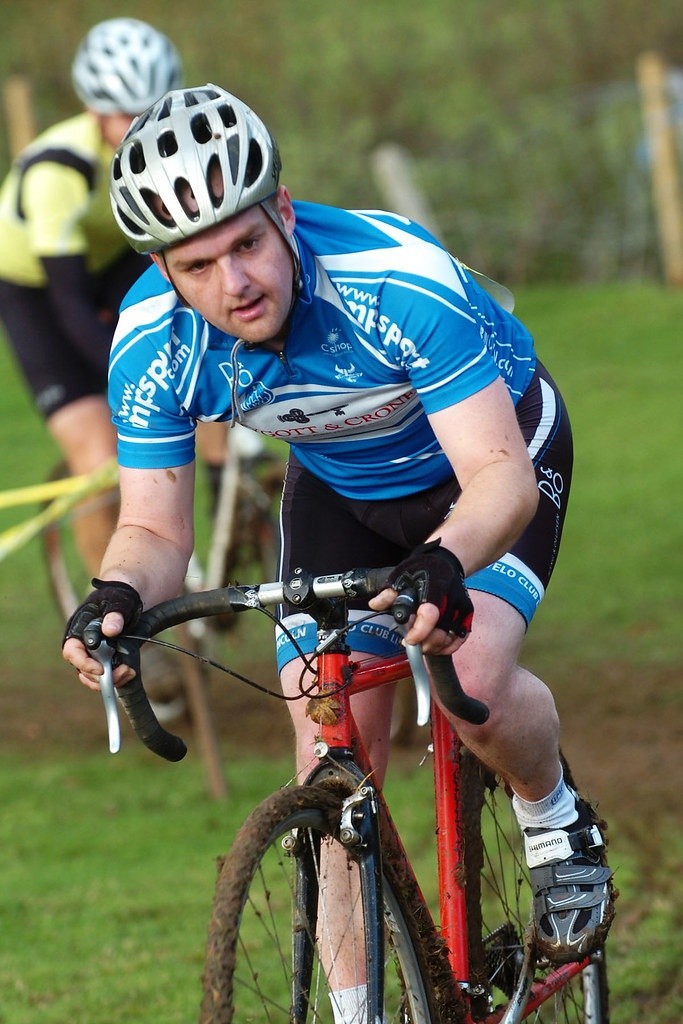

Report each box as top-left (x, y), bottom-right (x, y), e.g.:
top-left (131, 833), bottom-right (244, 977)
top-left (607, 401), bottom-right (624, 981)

top-left (520, 782), bottom-right (619, 963)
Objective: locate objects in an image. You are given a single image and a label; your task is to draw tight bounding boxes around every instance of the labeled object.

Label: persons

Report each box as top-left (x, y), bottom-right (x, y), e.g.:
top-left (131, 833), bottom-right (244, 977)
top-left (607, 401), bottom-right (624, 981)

top-left (60, 83), bottom-right (613, 1024)
top-left (0, 18), bottom-right (277, 714)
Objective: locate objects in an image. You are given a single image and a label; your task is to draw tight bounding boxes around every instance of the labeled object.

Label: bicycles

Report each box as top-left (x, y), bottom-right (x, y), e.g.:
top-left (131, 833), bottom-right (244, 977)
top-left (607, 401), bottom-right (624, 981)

top-left (85, 567), bottom-right (620, 1024)
top-left (40, 414), bottom-right (416, 743)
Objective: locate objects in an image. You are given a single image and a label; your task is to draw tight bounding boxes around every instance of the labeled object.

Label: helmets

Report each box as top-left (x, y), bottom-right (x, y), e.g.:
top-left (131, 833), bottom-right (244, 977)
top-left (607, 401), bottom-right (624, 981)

top-left (72, 17), bottom-right (182, 118)
top-left (108, 83), bottom-right (281, 255)
